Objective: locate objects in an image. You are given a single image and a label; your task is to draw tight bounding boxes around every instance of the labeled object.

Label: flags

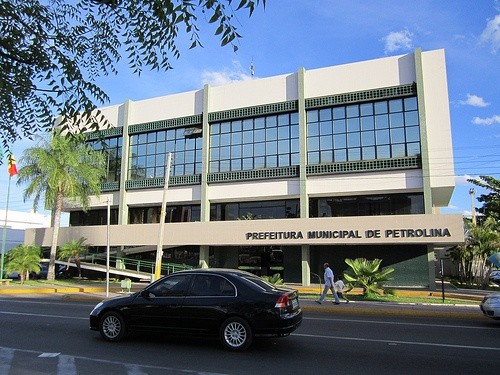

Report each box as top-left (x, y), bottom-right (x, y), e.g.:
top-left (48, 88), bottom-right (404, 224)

top-left (7, 155), bottom-right (18, 176)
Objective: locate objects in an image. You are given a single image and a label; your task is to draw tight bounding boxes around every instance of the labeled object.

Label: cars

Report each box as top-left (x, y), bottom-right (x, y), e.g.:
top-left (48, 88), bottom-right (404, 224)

top-left (89, 268), bottom-right (304, 353)
top-left (3, 265), bottom-right (48, 279)
top-left (489, 271), bottom-right (500, 281)
top-left (479, 294), bottom-right (500, 320)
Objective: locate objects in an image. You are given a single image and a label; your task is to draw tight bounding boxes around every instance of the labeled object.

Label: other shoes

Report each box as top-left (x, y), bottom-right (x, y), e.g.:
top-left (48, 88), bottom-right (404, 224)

top-left (333, 302), bottom-right (340, 304)
top-left (315, 300), bottom-right (321, 304)
top-left (345, 299), bottom-right (349, 303)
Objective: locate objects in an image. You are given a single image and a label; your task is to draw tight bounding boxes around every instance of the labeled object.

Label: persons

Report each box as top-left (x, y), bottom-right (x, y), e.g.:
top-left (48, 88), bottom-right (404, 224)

top-left (332, 274), bottom-right (349, 304)
top-left (315, 263), bottom-right (340, 305)
top-left (321, 213), bottom-right (330, 217)
top-left (286, 211), bottom-right (297, 218)
